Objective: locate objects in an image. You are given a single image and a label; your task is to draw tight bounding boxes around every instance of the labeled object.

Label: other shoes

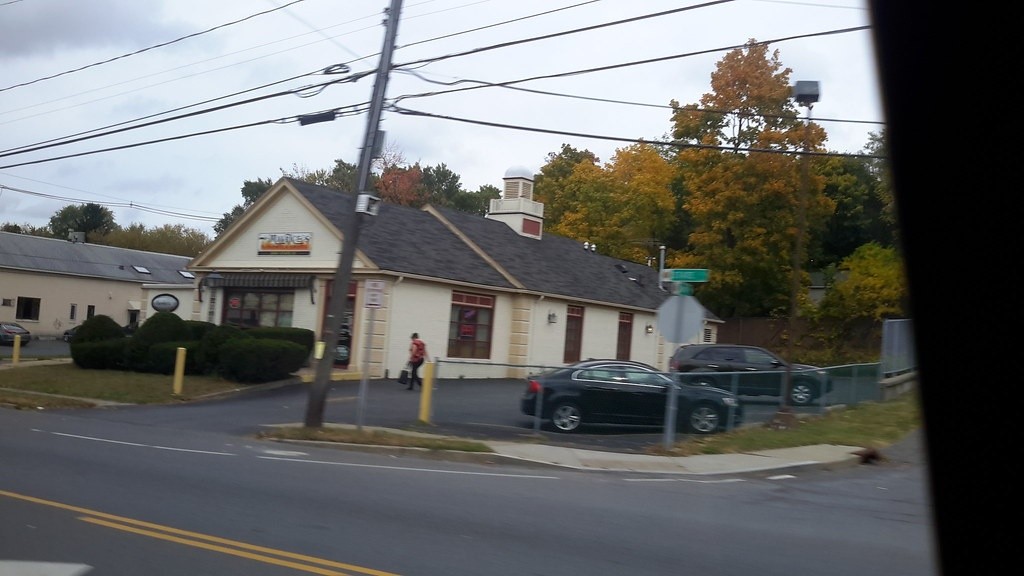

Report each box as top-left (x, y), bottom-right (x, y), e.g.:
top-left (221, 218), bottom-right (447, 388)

top-left (406, 387), bottom-right (412, 390)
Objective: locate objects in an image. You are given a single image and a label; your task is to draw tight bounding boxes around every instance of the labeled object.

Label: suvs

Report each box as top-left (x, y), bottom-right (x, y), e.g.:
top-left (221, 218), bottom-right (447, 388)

top-left (669, 343), bottom-right (835, 406)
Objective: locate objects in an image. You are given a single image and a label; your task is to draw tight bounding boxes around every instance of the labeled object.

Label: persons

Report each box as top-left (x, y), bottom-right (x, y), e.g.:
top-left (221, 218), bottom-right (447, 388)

top-left (406, 333), bottom-right (427, 391)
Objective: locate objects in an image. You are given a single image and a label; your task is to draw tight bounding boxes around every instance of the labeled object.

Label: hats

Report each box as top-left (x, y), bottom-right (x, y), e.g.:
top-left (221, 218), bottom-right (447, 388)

top-left (411, 333), bottom-right (418, 338)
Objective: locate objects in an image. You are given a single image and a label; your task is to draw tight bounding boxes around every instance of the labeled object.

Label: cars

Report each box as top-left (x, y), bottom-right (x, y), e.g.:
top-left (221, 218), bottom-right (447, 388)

top-left (64, 324), bottom-right (82, 344)
top-left (520, 357), bottom-right (744, 436)
top-left (0, 322), bottom-right (31, 346)
